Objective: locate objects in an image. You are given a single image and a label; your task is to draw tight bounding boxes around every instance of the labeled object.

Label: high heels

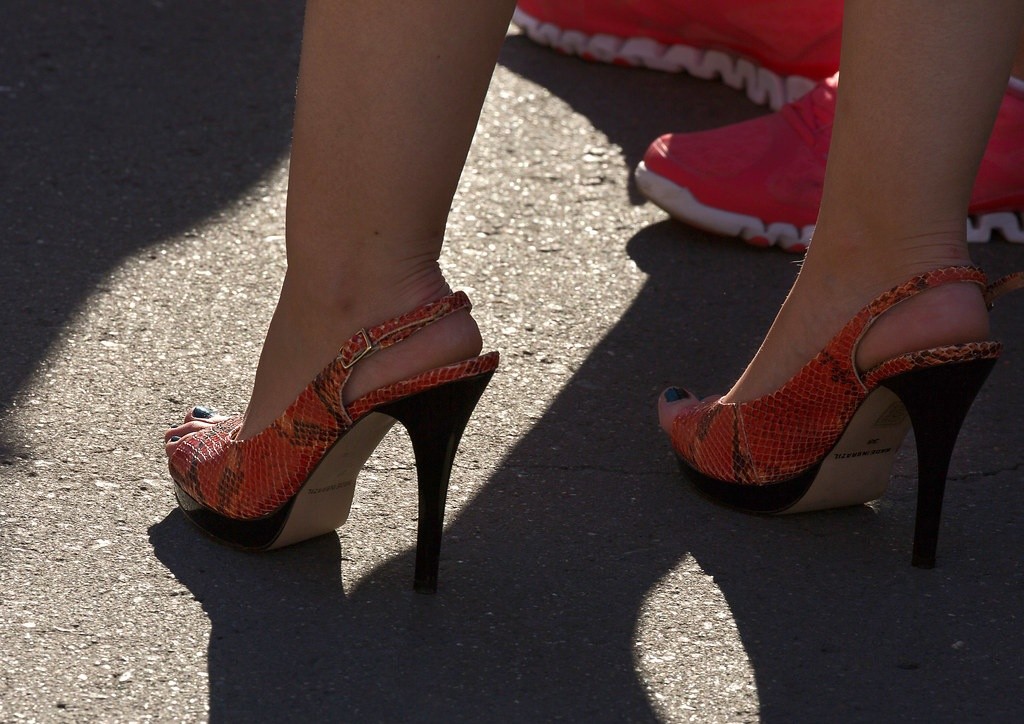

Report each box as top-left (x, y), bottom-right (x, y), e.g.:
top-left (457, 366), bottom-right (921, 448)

top-left (165, 290), bottom-right (500, 594)
top-left (658, 265), bottom-right (1024, 570)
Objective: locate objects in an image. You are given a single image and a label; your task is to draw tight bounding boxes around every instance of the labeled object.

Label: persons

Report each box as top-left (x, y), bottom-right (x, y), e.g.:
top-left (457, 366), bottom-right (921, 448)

top-left (164, 0), bottom-right (1024, 592)
top-left (511, 0), bottom-right (1024, 252)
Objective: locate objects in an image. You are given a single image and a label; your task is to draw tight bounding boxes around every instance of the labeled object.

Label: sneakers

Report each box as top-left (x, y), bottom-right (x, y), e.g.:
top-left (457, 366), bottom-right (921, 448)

top-left (633, 70), bottom-right (1024, 252)
top-left (512, 0), bottom-right (844, 113)
